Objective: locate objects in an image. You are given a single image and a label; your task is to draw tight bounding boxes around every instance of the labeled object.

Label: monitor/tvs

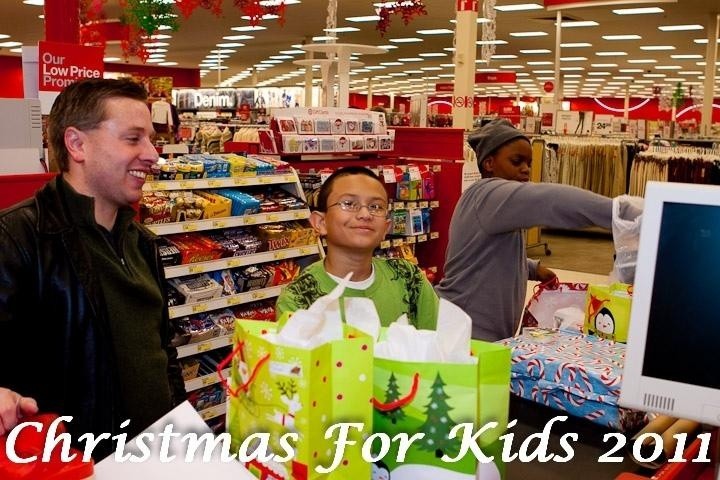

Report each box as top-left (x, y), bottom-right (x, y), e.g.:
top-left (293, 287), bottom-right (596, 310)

top-left (618, 179), bottom-right (720, 427)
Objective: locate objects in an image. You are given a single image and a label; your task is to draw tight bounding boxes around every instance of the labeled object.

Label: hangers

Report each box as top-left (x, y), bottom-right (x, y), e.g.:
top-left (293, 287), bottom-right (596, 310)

top-left (540, 137), bottom-right (719, 167)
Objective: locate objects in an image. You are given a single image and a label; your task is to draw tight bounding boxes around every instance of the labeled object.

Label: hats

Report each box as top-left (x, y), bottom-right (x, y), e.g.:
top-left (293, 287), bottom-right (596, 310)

top-left (466, 115), bottom-right (530, 169)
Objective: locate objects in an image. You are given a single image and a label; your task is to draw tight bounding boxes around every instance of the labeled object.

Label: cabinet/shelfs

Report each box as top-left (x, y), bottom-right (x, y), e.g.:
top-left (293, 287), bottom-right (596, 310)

top-left (140, 161), bottom-right (326, 422)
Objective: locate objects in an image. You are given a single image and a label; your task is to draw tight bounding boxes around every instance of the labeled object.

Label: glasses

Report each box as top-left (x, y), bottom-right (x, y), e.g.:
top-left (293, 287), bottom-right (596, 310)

top-left (325, 197), bottom-right (390, 219)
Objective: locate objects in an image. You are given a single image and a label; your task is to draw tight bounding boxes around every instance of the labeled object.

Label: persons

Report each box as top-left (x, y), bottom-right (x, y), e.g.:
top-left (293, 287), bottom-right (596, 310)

top-left (433, 119), bottom-right (641, 343)
top-left (0, 77), bottom-right (186, 463)
top-left (274, 165), bottom-right (438, 329)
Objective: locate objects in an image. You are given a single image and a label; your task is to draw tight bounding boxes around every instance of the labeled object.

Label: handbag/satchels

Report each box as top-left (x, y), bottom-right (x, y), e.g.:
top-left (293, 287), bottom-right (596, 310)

top-left (519, 277), bottom-right (587, 334)
top-left (590, 283), bottom-right (634, 342)
top-left (372, 326), bottom-right (512, 480)
top-left (216, 308), bottom-right (374, 478)
